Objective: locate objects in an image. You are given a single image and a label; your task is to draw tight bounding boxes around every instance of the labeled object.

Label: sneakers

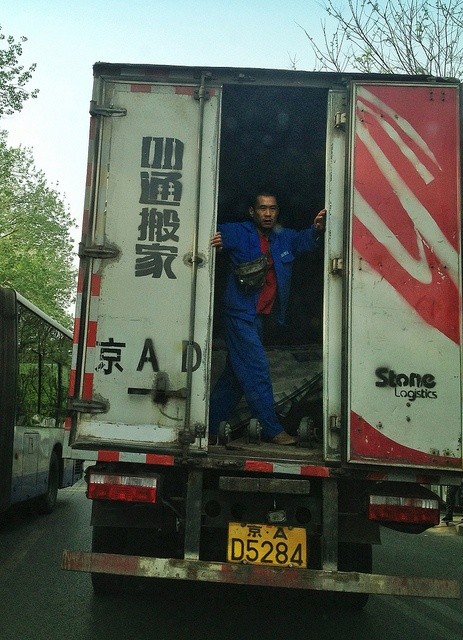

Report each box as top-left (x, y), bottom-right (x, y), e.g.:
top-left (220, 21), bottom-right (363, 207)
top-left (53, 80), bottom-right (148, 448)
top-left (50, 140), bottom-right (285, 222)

top-left (265, 431), bottom-right (296, 445)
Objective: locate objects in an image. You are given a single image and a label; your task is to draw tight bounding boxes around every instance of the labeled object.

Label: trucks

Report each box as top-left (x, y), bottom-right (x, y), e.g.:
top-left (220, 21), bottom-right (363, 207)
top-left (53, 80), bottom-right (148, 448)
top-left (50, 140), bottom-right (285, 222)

top-left (59, 60), bottom-right (463, 612)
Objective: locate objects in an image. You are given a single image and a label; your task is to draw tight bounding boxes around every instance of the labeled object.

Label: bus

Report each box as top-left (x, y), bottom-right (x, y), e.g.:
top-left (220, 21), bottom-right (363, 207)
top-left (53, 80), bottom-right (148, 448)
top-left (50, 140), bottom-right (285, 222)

top-left (0, 280), bottom-right (85, 515)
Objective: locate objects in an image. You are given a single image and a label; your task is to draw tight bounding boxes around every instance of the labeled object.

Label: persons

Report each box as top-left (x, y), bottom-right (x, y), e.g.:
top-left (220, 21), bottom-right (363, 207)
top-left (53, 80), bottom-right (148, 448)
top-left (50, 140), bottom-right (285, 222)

top-left (209, 191), bottom-right (328, 447)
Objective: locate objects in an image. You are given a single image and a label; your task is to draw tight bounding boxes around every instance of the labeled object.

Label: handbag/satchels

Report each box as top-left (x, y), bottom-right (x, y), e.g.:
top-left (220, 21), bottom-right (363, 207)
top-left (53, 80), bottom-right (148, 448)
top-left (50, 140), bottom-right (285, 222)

top-left (234, 256), bottom-right (268, 292)
